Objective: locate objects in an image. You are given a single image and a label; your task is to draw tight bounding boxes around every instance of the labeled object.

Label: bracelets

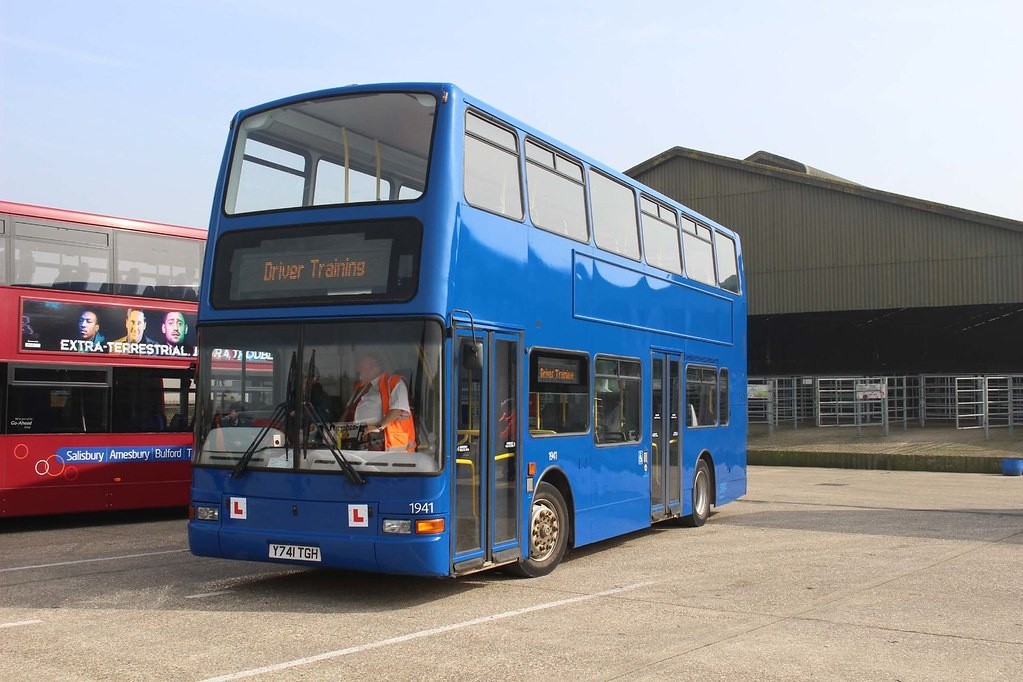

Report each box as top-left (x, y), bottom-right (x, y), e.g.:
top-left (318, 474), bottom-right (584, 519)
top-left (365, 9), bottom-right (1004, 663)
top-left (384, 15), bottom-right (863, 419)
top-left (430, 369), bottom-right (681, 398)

top-left (376, 424), bottom-right (382, 430)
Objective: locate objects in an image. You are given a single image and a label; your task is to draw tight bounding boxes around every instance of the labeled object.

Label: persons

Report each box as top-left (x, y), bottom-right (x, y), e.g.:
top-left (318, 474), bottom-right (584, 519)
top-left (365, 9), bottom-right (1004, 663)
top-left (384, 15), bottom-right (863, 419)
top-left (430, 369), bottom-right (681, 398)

top-left (230, 409), bottom-right (241, 426)
top-left (78, 310), bottom-right (105, 352)
top-left (339, 353), bottom-right (417, 453)
top-left (111, 309), bottom-right (159, 355)
top-left (159, 312), bottom-right (194, 357)
top-left (290, 364), bottom-right (333, 421)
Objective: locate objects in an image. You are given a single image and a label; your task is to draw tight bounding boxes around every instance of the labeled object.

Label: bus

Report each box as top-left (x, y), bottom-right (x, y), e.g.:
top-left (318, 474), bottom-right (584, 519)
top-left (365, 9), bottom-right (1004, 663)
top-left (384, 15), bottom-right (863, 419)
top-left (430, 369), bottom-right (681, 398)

top-left (0, 200), bottom-right (274, 518)
top-left (188, 83), bottom-right (746, 579)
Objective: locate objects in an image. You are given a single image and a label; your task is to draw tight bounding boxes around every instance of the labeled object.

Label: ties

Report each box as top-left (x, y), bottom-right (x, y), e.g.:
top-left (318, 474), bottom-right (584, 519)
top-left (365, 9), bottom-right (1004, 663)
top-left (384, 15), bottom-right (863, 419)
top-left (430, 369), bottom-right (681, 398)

top-left (347, 382), bottom-right (372, 422)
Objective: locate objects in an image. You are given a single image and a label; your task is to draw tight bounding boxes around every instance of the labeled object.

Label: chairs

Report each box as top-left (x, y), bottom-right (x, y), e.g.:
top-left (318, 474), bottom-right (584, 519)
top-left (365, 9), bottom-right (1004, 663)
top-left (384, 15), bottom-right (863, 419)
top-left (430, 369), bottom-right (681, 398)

top-left (142, 413), bottom-right (195, 432)
top-left (14, 255), bottom-right (200, 302)
